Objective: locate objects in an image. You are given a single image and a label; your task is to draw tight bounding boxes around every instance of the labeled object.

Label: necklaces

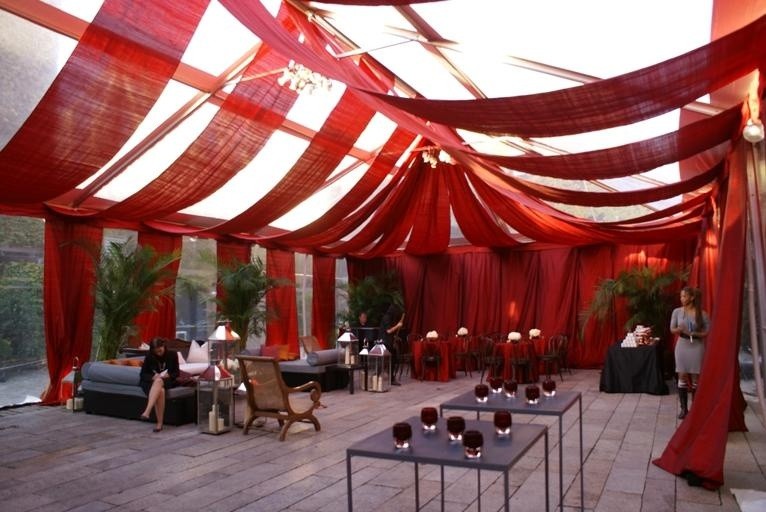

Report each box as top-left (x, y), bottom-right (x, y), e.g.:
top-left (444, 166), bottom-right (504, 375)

top-left (158, 360), bottom-right (166, 372)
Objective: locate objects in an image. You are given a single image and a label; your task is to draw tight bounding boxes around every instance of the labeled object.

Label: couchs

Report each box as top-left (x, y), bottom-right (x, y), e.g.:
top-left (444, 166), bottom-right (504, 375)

top-left (78, 355), bottom-right (197, 428)
top-left (242, 346), bottom-right (347, 392)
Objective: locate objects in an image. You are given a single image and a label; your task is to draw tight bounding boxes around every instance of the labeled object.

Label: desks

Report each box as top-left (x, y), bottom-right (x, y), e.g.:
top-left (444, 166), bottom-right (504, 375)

top-left (346, 383), bottom-right (583, 512)
top-left (606, 337), bottom-right (663, 395)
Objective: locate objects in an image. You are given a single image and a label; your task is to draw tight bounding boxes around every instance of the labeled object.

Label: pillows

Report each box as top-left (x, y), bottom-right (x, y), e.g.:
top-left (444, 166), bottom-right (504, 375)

top-left (260, 344), bottom-right (298, 360)
top-left (107, 359), bottom-right (143, 367)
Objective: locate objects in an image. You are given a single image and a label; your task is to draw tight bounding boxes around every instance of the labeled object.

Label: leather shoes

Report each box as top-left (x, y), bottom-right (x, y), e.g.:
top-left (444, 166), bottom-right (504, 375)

top-left (141, 413), bottom-right (150, 420)
top-left (153, 426), bottom-right (163, 432)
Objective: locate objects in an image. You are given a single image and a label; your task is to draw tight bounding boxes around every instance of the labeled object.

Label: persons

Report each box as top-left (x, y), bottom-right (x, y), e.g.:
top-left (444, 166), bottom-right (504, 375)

top-left (380, 303), bottom-right (403, 386)
top-left (352, 310), bottom-right (379, 351)
top-left (670, 287), bottom-right (711, 419)
top-left (139, 337), bottom-right (180, 432)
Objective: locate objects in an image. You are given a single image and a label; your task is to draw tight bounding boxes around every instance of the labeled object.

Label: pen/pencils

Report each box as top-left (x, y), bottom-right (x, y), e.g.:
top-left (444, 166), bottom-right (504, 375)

top-left (152, 369), bottom-right (158, 374)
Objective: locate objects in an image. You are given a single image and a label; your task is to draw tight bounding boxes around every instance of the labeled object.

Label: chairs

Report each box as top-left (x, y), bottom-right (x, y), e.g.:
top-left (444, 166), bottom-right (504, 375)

top-left (388, 329), bottom-right (569, 388)
top-left (235, 356), bottom-right (321, 444)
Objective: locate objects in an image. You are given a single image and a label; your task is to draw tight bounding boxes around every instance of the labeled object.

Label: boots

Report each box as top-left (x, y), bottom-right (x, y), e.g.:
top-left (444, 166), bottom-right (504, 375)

top-left (691, 388), bottom-right (696, 402)
top-left (678, 387), bottom-right (689, 419)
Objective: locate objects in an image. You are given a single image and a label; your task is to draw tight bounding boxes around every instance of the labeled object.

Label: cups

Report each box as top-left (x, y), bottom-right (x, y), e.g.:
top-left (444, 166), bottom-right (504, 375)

top-left (494, 410), bottom-right (512, 441)
top-left (475, 384), bottom-right (489, 403)
top-left (490, 377), bottom-right (518, 398)
top-left (420, 407), bottom-right (438, 432)
top-left (542, 380), bottom-right (557, 397)
top-left (392, 423), bottom-right (412, 449)
top-left (525, 386), bottom-right (540, 405)
top-left (445, 416), bottom-right (484, 459)
